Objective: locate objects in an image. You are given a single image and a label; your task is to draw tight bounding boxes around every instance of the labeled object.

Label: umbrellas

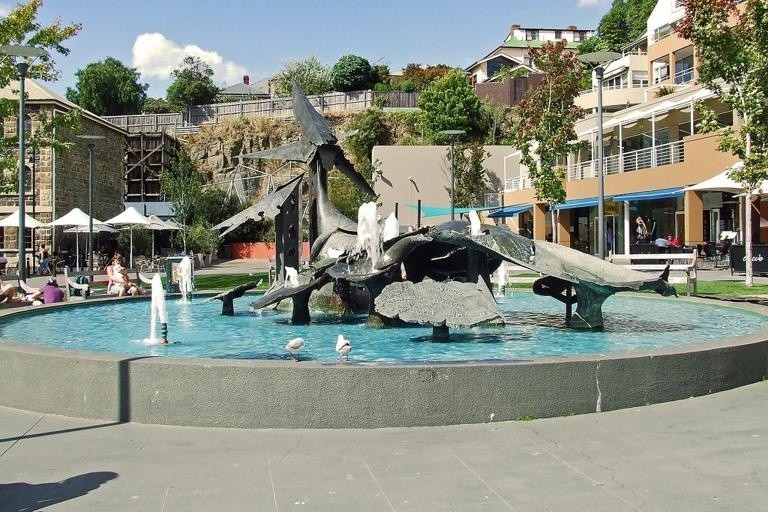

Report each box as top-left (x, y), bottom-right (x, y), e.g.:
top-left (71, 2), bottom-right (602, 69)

top-left (673, 160), bottom-right (767, 244)
top-left (731, 176), bottom-right (768, 199)
top-left (0, 206), bottom-right (190, 268)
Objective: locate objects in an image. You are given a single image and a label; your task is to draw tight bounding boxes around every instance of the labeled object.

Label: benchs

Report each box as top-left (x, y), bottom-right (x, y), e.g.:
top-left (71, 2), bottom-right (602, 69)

top-left (604, 248), bottom-right (698, 297)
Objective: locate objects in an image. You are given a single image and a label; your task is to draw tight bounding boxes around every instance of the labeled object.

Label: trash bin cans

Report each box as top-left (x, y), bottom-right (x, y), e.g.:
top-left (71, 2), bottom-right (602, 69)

top-left (629, 244), bottom-right (659, 265)
top-left (731, 243), bottom-right (768, 274)
top-left (165, 256), bottom-right (194, 292)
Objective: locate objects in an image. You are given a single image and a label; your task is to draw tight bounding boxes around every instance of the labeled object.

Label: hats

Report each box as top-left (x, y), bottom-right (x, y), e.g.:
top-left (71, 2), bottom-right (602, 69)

top-left (46, 277), bottom-right (57, 283)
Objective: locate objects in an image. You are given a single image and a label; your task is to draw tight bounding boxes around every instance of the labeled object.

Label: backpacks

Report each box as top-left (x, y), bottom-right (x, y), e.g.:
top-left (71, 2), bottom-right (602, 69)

top-left (71, 275), bottom-right (90, 296)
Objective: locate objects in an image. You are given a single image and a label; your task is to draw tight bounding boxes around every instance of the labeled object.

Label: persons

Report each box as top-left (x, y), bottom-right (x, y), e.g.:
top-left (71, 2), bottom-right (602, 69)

top-left (635, 219), bottom-right (650, 245)
top-left (415, 223), bottom-right (424, 230)
top-left (38, 243), bottom-right (53, 276)
top-left (702, 240), bottom-right (716, 256)
top-left (654, 233), bottom-right (669, 247)
top-left (112, 264), bottom-right (145, 294)
top-left (666, 236), bottom-right (681, 248)
top-left (407, 225), bottom-right (415, 232)
top-left (104, 253), bottom-right (138, 297)
top-left (0, 251), bottom-right (8, 276)
top-left (27, 278), bottom-right (64, 304)
top-left (0, 278), bottom-right (21, 304)
top-left (635, 215), bottom-right (643, 244)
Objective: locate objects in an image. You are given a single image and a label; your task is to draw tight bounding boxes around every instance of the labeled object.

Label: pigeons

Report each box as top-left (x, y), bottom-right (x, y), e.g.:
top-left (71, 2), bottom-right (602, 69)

top-left (334, 334), bottom-right (352, 362)
top-left (282, 337), bottom-right (305, 362)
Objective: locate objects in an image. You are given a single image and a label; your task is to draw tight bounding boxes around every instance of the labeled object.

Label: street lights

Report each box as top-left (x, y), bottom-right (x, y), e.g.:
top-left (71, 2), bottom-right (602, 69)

top-left (78, 135), bottom-right (107, 282)
top-left (577, 50), bottom-right (622, 260)
top-left (439, 129), bottom-right (465, 221)
top-left (0, 45), bottom-right (50, 285)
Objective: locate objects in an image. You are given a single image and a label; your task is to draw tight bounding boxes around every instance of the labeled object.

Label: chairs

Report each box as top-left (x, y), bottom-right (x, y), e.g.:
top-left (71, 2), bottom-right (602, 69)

top-left (135, 254), bottom-right (167, 274)
top-left (0, 266), bottom-right (142, 307)
top-left (681, 240), bottom-right (732, 271)
